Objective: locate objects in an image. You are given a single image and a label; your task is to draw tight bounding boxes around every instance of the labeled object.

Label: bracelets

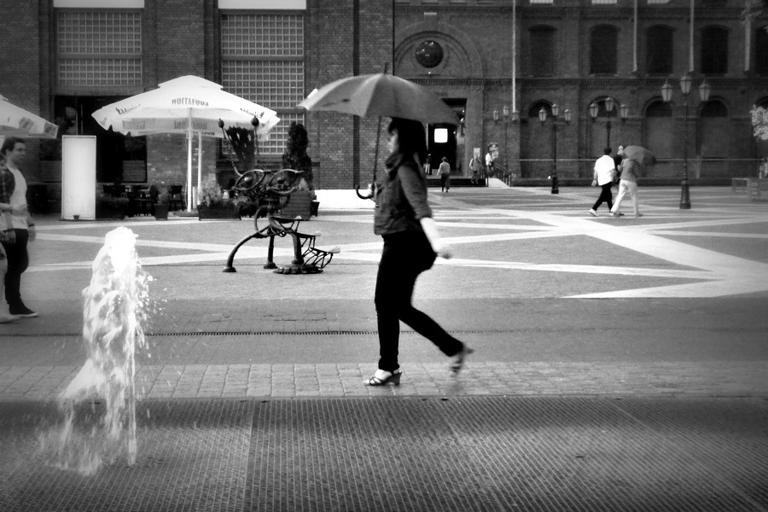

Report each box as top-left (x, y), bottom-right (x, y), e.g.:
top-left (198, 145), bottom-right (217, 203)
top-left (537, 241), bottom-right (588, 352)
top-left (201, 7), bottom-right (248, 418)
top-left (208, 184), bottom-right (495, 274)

top-left (7, 228), bottom-right (14, 232)
top-left (29, 223), bottom-right (34, 227)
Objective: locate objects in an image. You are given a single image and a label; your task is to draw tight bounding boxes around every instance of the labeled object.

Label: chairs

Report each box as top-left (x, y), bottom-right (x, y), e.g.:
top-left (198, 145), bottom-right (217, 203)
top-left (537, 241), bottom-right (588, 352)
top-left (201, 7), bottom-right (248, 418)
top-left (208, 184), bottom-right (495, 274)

top-left (102, 182), bottom-right (187, 220)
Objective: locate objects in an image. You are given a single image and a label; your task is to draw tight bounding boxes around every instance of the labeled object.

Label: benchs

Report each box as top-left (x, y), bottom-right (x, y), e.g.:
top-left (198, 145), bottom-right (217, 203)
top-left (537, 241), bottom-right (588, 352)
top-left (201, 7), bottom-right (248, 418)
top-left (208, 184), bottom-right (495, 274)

top-left (731, 177), bottom-right (768, 202)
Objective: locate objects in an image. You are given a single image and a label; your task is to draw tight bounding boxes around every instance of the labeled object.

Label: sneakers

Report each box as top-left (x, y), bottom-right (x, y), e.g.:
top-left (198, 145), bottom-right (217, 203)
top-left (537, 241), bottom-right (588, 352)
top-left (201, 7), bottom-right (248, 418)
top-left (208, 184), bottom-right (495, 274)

top-left (589, 208), bottom-right (597, 217)
top-left (9, 299), bottom-right (37, 317)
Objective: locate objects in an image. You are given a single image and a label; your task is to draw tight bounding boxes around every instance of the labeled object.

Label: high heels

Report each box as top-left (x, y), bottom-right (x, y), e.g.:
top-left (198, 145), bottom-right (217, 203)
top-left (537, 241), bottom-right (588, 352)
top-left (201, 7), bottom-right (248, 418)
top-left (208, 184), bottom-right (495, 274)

top-left (364, 368), bottom-right (402, 386)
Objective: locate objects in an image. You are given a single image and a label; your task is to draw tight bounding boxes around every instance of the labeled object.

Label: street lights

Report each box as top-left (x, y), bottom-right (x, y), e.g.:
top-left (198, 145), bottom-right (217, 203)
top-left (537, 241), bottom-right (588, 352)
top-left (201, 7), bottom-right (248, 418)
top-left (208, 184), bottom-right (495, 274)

top-left (537, 104), bottom-right (571, 193)
top-left (588, 96), bottom-right (627, 148)
top-left (659, 69), bottom-right (711, 209)
top-left (492, 105), bottom-right (519, 170)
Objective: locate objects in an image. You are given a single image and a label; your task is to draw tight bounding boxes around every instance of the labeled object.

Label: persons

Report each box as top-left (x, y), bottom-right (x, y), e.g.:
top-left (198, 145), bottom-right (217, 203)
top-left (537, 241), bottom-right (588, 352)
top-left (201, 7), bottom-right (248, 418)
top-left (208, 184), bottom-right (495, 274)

top-left (485, 151), bottom-right (492, 172)
top-left (436, 157), bottom-right (450, 193)
top-left (610, 158), bottom-right (645, 217)
top-left (0, 136), bottom-right (38, 319)
top-left (0, 155), bottom-right (27, 323)
top-left (0, 202), bottom-right (27, 323)
top-left (613, 150), bottom-right (624, 186)
top-left (589, 147), bottom-right (617, 217)
top-left (469, 154), bottom-right (482, 184)
top-left (361, 118), bottom-right (473, 386)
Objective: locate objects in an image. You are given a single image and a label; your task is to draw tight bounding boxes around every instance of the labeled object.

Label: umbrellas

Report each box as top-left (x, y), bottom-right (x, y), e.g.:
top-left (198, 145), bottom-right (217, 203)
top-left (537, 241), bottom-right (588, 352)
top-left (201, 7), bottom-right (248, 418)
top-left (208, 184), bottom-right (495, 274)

top-left (92, 72), bottom-right (275, 212)
top-left (0, 96), bottom-right (58, 140)
top-left (296, 61), bottom-right (459, 199)
top-left (149, 115), bottom-right (281, 205)
top-left (624, 144), bottom-right (657, 167)
